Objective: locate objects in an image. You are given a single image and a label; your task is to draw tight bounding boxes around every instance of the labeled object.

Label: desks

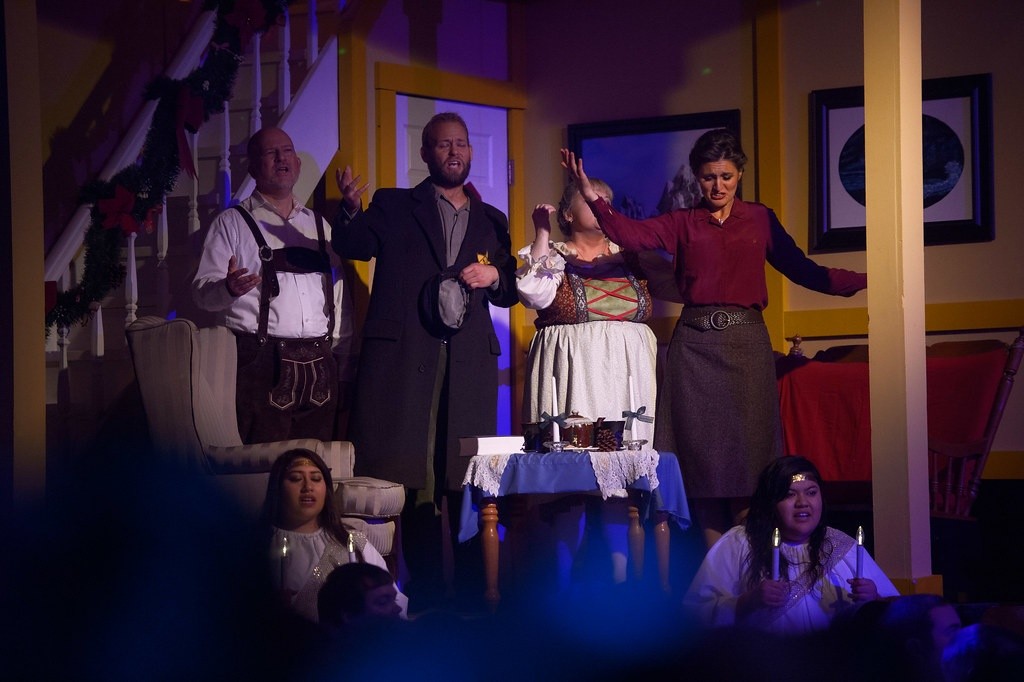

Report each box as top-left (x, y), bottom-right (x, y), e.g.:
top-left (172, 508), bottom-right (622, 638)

top-left (774, 338), bottom-right (1011, 512)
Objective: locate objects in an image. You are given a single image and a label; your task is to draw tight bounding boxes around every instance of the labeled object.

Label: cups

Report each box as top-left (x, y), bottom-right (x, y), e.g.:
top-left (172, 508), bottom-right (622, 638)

top-left (520, 420), bottom-right (627, 454)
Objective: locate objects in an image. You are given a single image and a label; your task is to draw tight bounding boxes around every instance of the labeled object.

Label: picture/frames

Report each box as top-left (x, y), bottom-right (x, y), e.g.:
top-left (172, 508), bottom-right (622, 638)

top-left (811, 71), bottom-right (996, 249)
top-left (565, 107), bottom-right (746, 216)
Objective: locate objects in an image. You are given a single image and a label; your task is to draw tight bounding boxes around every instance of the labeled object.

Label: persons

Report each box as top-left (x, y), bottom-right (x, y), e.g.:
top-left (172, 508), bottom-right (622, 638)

top-left (321, 563), bottom-right (402, 639)
top-left (245, 448), bottom-right (409, 626)
top-left (334, 113), bottom-right (515, 621)
top-left (516, 178), bottom-right (655, 611)
top-left (190, 127), bottom-right (344, 450)
top-left (687, 456), bottom-right (903, 680)
top-left (555, 133), bottom-right (868, 551)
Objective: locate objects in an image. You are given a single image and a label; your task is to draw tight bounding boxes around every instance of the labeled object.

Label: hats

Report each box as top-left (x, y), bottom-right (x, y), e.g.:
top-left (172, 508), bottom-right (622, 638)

top-left (419, 266), bottom-right (484, 337)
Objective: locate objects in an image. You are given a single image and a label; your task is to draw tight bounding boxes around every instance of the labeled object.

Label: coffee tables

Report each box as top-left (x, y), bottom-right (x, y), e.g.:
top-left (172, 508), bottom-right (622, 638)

top-left (453, 451), bottom-right (687, 606)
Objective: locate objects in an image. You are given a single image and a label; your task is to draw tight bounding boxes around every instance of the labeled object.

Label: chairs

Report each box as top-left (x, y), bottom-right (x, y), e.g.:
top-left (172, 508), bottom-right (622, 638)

top-left (125, 317), bottom-right (403, 601)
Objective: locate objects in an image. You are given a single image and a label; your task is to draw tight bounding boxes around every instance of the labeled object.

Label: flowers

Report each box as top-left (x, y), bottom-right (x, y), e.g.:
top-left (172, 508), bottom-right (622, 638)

top-left (45, 26), bottom-right (246, 337)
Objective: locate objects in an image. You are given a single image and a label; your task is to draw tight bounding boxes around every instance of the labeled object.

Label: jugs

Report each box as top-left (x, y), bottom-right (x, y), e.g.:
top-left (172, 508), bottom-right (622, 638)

top-left (556, 411), bottom-right (606, 447)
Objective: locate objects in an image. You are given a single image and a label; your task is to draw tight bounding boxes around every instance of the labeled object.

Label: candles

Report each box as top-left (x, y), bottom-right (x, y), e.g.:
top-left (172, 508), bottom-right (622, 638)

top-left (770, 528), bottom-right (782, 579)
top-left (855, 525), bottom-right (866, 579)
